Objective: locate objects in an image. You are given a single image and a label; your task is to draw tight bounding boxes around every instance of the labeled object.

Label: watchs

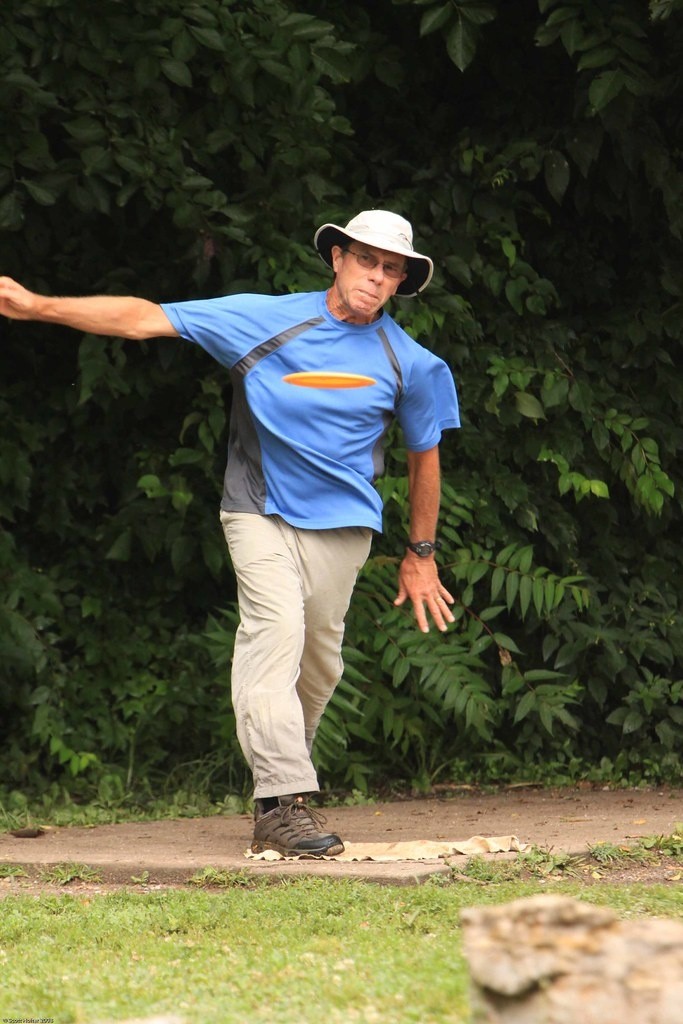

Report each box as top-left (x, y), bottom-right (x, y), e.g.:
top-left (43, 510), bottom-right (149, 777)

top-left (409, 539), bottom-right (436, 558)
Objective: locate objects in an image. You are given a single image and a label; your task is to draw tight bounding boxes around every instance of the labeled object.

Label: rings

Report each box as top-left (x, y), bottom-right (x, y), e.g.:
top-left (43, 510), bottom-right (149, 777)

top-left (436, 598), bottom-right (442, 601)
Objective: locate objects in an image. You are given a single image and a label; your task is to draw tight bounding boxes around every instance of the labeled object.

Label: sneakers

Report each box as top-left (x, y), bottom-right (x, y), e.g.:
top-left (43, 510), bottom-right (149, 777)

top-left (251, 793), bottom-right (346, 857)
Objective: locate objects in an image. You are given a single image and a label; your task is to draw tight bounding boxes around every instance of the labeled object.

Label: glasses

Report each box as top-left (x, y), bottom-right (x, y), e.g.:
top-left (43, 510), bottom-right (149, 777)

top-left (345, 249), bottom-right (404, 278)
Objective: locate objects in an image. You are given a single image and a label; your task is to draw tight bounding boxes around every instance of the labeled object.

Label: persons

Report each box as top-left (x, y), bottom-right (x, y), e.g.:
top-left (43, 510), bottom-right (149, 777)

top-left (0, 208), bottom-right (460, 856)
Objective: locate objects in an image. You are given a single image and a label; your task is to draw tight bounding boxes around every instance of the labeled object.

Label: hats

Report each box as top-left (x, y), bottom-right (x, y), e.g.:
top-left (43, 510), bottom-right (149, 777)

top-left (314, 209), bottom-right (434, 298)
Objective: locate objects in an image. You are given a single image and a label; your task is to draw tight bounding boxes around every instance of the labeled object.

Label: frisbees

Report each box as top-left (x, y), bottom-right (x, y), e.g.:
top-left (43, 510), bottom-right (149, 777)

top-left (281, 371), bottom-right (378, 390)
